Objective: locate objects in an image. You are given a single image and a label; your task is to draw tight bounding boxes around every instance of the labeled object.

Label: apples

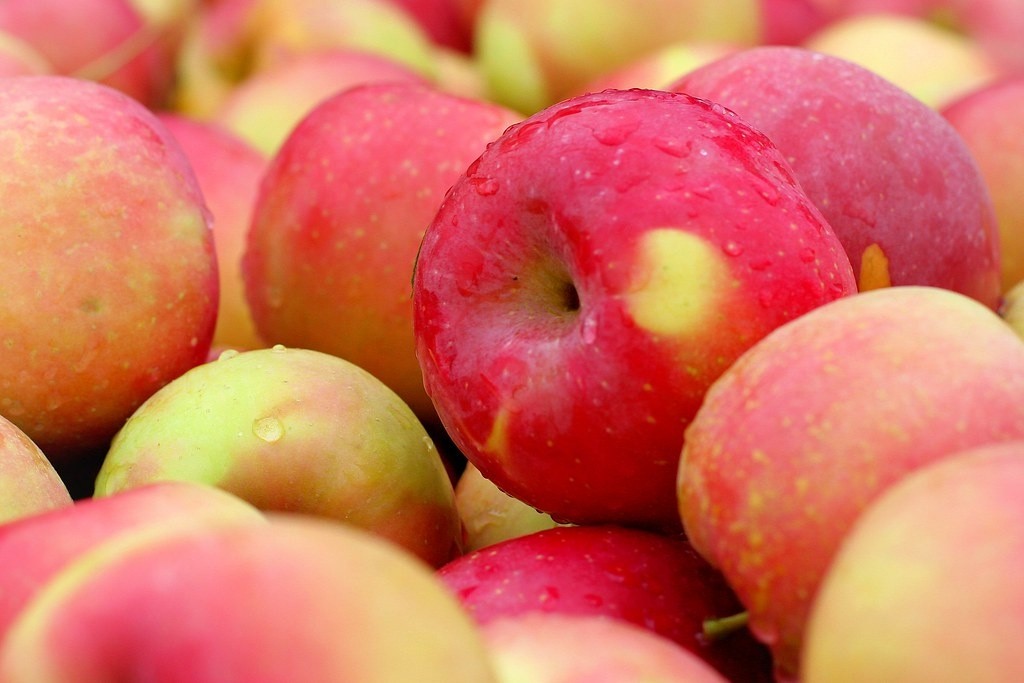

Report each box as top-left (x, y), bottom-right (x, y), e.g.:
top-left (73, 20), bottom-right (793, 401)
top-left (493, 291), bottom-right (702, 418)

top-left (0, 0), bottom-right (1024, 683)
